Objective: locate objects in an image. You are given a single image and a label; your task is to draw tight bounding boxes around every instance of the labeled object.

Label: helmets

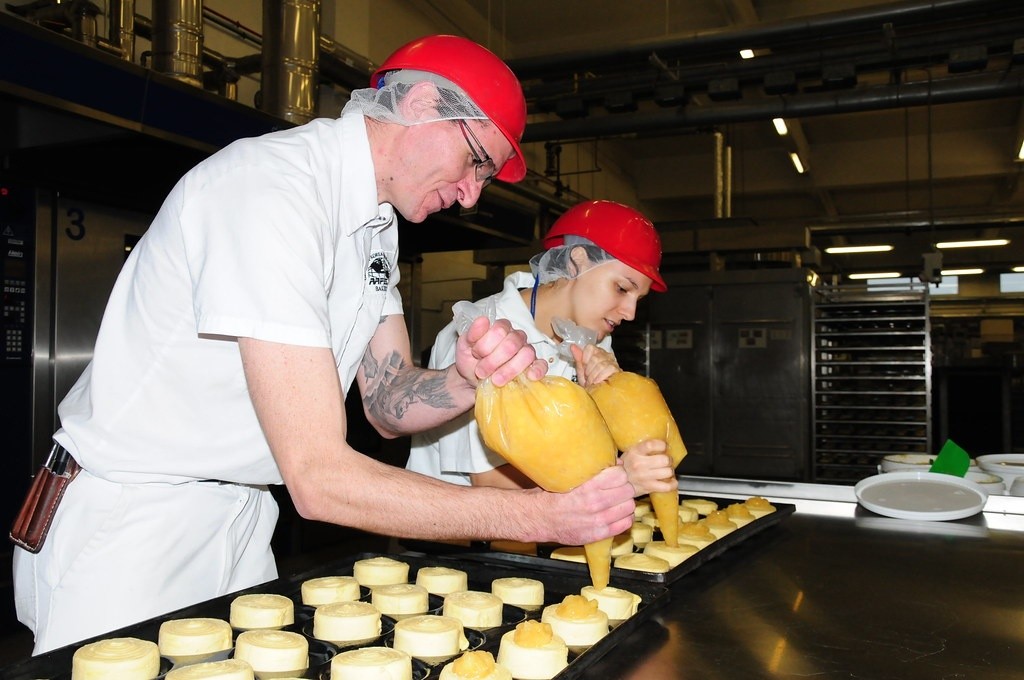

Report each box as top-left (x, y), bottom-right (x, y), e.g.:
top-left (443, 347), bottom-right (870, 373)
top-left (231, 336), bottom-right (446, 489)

top-left (369, 33), bottom-right (530, 186)
top-left (542, 199), bottom-right (668, 294)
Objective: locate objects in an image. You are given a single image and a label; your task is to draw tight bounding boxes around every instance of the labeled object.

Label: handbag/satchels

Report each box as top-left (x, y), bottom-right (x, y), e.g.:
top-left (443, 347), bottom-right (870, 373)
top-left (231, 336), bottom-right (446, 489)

top-left (10, 441), bottom-right (83, 554)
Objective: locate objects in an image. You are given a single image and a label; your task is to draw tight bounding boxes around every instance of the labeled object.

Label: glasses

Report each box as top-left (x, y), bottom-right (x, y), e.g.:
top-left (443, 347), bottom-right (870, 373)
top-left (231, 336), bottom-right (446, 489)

top-left (457, 119), bottom-right (496, 189)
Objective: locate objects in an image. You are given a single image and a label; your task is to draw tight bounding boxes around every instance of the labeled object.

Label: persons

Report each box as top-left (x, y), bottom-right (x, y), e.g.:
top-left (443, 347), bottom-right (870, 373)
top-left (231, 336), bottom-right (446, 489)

top-left (12, 36), bottom-right (636, 657)
top-left (389, 198), bottom-right (667, 549)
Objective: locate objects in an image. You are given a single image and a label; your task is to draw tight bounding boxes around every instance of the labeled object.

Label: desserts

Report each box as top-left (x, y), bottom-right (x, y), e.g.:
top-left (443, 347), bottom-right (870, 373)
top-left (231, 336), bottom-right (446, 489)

top-left (72, 497), bottom-right (777, 680)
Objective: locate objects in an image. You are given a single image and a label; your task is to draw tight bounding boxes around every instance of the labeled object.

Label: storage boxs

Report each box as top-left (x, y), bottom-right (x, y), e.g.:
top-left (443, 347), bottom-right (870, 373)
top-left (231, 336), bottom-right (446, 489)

top-left (982, 335), bottom-right (1014, 342)
top-left (980, 319), bottom-right (1014, 335)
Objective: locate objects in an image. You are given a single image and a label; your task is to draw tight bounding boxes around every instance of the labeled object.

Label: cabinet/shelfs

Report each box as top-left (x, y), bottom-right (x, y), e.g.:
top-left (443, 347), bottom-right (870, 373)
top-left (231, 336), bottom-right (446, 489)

top-left (808, 280), bottom-right (933, 486)
